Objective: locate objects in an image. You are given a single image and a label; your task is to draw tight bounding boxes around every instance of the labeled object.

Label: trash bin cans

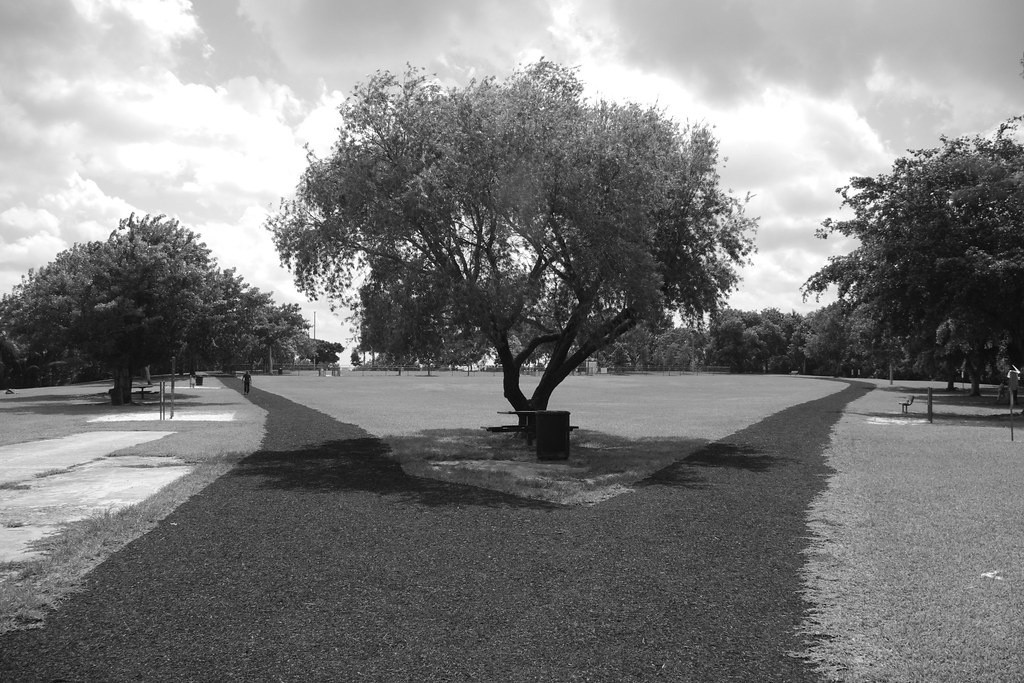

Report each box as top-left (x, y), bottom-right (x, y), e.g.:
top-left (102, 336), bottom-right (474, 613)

top-left (532, 410), bottom-right (571, 461)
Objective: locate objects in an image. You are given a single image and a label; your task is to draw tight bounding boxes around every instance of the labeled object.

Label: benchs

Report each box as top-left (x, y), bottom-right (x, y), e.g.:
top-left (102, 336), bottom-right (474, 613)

top-left (898, 394), bottom-right (915, 414)
top-left (131, 391), bottom-right (160, 395)
top-left (481, 425), bottom-right (580, 446)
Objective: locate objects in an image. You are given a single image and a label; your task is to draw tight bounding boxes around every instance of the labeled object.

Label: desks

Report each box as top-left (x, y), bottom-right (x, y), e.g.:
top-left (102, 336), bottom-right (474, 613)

top-left (497, 409), bottom-right (547, 424)
top-left (131, 385), bottom-right (154, 399)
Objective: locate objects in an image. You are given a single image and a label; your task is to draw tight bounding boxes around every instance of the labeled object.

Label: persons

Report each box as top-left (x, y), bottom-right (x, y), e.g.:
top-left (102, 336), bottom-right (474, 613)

top-left (189, 367), bottom-right (196, 388)
top-left (241, 371), bottom-right (252, 395)
top-left (1008, 363), bottom-right (1021, 407)
